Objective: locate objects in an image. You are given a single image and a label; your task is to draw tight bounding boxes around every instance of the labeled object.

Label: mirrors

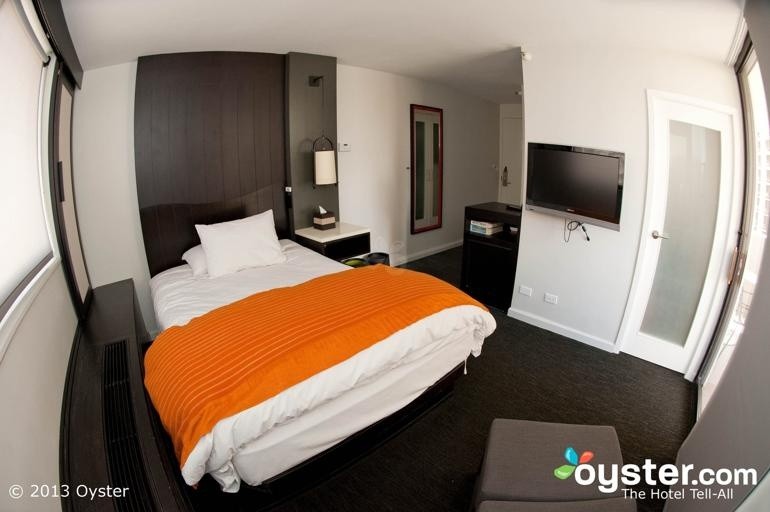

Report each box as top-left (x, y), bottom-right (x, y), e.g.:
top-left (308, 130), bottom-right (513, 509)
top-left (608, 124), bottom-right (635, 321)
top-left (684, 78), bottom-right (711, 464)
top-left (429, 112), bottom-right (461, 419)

top-left (409, 103), bottom-right (444, 235)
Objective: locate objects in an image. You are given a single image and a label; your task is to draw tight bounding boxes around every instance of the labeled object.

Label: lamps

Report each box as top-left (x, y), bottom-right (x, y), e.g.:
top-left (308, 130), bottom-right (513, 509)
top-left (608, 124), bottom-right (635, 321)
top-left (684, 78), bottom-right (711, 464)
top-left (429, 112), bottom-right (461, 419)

top-left (308, 74), bottom-right (339, 191)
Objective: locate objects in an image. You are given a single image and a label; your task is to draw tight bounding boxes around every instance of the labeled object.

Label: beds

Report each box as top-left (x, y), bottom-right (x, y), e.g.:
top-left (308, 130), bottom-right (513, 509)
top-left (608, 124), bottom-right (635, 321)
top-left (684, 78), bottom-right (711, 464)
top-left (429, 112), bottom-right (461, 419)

top-left (147, 237), bottom-right (499, 511)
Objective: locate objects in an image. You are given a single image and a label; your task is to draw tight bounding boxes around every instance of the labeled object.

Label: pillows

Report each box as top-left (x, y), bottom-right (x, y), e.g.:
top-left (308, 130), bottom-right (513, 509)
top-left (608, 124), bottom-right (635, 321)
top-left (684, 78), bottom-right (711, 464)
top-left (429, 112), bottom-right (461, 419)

top-left (180, 208), bottom-right (287, 280)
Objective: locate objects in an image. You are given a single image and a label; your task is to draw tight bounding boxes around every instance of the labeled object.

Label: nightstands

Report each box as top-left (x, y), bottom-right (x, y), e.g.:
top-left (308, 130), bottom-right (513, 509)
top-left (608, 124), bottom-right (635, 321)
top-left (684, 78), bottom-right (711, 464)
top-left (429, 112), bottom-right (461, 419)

top-left (294, 221), bottom-right (371, 262)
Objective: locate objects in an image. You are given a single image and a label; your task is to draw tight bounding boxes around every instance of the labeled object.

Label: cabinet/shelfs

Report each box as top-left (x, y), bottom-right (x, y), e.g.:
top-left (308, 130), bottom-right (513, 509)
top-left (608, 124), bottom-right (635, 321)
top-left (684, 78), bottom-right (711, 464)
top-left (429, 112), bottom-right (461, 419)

top-left (459, 200), bottom-right (523, 315)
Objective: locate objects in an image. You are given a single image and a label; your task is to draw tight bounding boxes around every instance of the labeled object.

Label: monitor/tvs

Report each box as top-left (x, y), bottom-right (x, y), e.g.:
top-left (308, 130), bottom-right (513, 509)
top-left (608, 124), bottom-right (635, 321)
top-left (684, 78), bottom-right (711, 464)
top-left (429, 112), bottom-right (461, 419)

top-left (525, 142), bottom-right (624, 232)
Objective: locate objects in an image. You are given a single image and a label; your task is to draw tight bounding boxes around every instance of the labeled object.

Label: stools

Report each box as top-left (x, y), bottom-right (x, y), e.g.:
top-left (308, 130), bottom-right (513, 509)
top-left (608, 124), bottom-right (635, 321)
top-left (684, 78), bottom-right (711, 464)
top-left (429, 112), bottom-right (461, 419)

top-left (473, 416), bottom-right (627, 500)
